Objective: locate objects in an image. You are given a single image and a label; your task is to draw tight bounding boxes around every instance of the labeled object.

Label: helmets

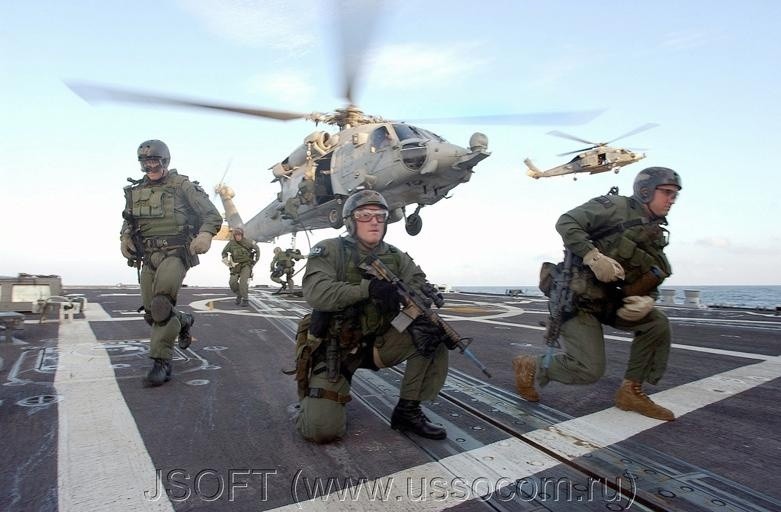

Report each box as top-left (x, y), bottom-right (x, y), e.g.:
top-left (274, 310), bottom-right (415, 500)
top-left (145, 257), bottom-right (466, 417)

top-left (633, 167), bottom-right (682, 204)
top-left (233, 228), bottom-right (244, 237)
top-left (137, 140), bottom-right (170, 167)
top-left (342, 190), bottom-right (388, 236)
top-left (274, 247), bottom-right (282, 255)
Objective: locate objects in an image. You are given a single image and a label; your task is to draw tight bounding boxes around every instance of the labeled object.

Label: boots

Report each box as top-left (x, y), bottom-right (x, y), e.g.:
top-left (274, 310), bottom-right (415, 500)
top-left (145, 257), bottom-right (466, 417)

top-left (513, 355), bottom-right (540, 402)
top-left (242, 298), bottom-right (248, 307)
top-left (282, 281), bottom-right (286, 289)
top-left (391, 399), bottom-right (446, 439)
top-left (615, 378), bottom-right (674, 421)
top-left (147, 359), bottom-right (172, 385)
top-left (235, 295), bottom-right (241, 304)
top-left (178, 314), bottom-right (194, 349)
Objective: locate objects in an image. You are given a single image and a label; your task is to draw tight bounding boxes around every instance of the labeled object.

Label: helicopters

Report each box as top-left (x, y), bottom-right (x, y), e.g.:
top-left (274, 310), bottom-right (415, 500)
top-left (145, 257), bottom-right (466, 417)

top-left (63, 0), bottom-right (492, 245)
top-left (523, 129), bottom-right (644, 179)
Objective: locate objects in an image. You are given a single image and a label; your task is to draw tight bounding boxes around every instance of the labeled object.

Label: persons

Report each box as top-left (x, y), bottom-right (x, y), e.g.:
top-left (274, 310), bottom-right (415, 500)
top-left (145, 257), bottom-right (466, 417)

top-left (221, 228), bottom-right (260, 307)
top-left (292, 188), bottom-right (455, 443)
top-left (118, 138), bottom-right (223, 387)
top-left (512, 166), bottom-right (681, 421)
top-left (269, 246), bottom-right (304, 294)
top-left (278, 174), bottom-right (322, 222)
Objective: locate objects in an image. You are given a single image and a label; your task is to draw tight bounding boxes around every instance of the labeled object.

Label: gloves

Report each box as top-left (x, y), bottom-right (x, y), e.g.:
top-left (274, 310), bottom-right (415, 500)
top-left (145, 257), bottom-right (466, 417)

top-left (222, 257), bottom-right (232, 268)
top-left (120, 233), bottom-right (136, 259)
top-left (369, 277), bottom-right (398, 299)
top-left (582, 249), bottom-right (625, 282)
top-left (406, 316), bottom-right (449, 359)
top-left (616, 295), bottom-right (654, 321)
top-left (190, 232), bottom-right (213, 255)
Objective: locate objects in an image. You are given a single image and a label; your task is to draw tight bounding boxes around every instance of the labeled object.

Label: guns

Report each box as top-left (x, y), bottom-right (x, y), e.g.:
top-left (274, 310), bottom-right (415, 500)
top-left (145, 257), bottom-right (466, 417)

top-left (121, 184), bottom-right (141, 282)
top-left (541, 246), bottom-right (582, 369)
top-left (360, 252), bottom-right (493, 379)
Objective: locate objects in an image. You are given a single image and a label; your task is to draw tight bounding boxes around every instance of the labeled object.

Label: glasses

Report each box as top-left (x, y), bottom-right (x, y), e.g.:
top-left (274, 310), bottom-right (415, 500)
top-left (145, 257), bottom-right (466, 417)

top-left (656, 188), bottom-right (679, 198)
top-left (138, 156), bottom-right (167, 172)
top-left (350, 209), bottom-right (391, 223)
